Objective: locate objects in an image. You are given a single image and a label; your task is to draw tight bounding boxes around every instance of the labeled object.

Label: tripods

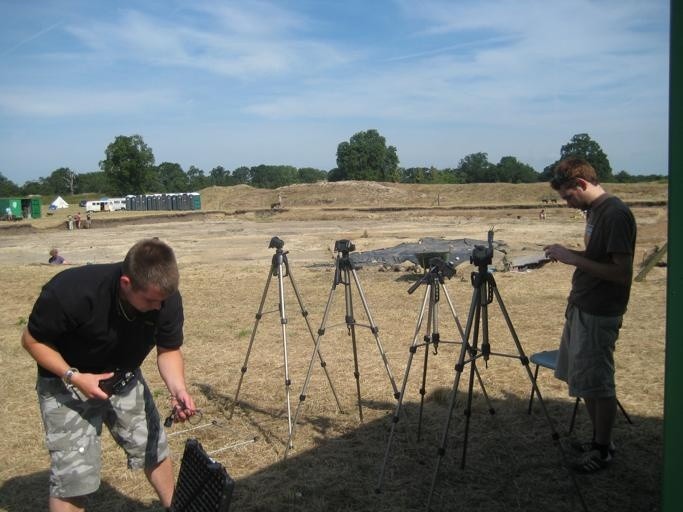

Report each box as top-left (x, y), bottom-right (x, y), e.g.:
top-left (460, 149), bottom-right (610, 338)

top-left (285, 252), bottom-right (416, 450)
top-left (229, 249), bottom-right (345, 450)
top-left (424, 273), bottom-right (590, 512)
top-left (374, 268), bottom-right (495, 494)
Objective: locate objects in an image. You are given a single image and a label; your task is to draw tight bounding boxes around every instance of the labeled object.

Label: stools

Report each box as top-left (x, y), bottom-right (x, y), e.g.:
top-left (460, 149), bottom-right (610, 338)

top-left (527, 350), bottom-right (633, 434)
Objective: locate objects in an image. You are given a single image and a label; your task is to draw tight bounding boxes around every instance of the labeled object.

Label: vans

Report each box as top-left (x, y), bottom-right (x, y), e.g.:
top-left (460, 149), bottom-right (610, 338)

top-left (84, 196), bottom-right (126, 213)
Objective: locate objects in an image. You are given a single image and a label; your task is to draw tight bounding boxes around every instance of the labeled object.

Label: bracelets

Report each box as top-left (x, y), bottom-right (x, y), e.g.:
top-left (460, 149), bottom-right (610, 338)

top-left (62, 366), bottom-right (79, 385)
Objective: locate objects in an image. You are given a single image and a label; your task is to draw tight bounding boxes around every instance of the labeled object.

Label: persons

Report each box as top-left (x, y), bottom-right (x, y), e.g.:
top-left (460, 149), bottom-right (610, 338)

top-left (85, 211), bottom-right (92, 229)
top-left (20, 240), bottom-right (198, 512)
top-left (73, 212), bottom-right (81, 229)
top-left (540, 153), bottom-right (637, 473)
top-left (6, 210), bottom-right (12, 221)
top-left (47, 248), bottom-right (67, 265)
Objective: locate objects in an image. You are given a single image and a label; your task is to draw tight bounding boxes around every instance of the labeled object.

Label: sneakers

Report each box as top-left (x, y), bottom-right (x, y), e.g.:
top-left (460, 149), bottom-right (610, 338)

top-left (572, 440), bottom-right (615, 473)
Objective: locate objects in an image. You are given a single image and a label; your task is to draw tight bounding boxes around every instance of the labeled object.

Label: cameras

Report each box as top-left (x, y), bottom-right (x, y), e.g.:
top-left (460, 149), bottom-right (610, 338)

top-left (98, 368), bottom-right (137, 395)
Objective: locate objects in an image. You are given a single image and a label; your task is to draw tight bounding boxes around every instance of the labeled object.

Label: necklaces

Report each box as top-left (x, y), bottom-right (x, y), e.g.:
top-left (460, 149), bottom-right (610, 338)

top-left (118, 299), bottom-right (138, 322)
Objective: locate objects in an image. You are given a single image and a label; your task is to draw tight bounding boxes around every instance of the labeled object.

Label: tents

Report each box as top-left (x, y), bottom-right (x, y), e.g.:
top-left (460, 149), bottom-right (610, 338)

top-left (51, 196), bottom-right (69, 209)
